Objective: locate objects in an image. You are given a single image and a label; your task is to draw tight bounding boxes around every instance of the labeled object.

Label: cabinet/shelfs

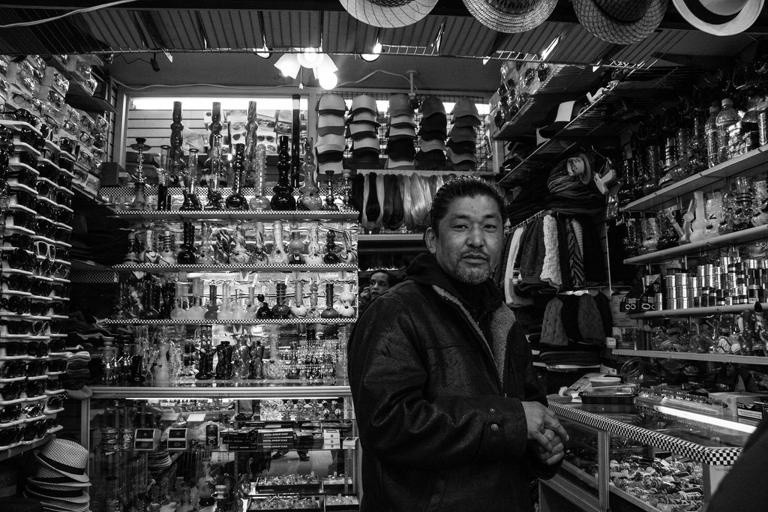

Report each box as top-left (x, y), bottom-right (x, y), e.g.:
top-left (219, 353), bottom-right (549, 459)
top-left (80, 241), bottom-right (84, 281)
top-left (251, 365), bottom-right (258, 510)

top-left (95, 204), bottom-right (362, 328)
top-left (591, 89), bottom-right (768, 373)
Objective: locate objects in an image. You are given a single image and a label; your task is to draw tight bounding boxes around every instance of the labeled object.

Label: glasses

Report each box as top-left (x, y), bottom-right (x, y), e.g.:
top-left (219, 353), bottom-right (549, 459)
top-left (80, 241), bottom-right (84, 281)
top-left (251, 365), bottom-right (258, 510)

top-left (1, 54), bottom-right (110, 195)
top-left (0, 196), bottom-right (69, 449)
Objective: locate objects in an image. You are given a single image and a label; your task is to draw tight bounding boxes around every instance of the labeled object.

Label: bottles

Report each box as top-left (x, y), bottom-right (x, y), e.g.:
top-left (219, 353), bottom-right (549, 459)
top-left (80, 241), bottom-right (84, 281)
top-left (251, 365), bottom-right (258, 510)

top-left (704, 97), bottom-right (738, 164)
top-left (117, 219), bottom-right (357, 266)
top-left (96, 324), bottom-right (350, 385)
top-left (111, 276), bottom-right (357, 319)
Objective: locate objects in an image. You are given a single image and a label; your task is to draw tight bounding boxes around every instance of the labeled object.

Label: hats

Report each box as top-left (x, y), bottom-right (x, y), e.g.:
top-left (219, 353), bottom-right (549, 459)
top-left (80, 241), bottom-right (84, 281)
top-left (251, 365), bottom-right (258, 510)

top-left (314, 93), bottom-right (482, 175)
top-left (566, 153), bottom-right (591, 185)
top-left (23, 438), bottom-right (91, 512)
top-left (339, 1), bottom-right (438, 28)
top-left (571, 0), bottom-right (669, 46)
top-left (462, 0), bottom-right (559, 34)
top-left (539, 292), bottom-right (613, 346)
top-left (672, 0), bottom-right (765, 37)
top-left (538, 100), bottom-right (585, 139)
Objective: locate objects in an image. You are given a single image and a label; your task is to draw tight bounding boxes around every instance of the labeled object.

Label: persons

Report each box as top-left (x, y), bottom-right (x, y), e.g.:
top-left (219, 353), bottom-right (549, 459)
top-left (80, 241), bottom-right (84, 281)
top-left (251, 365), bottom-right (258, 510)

top-left (345, 174), bottom-right (572, 512)
top-left (706, 415), bottom-right (768, 511)
top-left (360, 269), bottom-right (399, 305)
